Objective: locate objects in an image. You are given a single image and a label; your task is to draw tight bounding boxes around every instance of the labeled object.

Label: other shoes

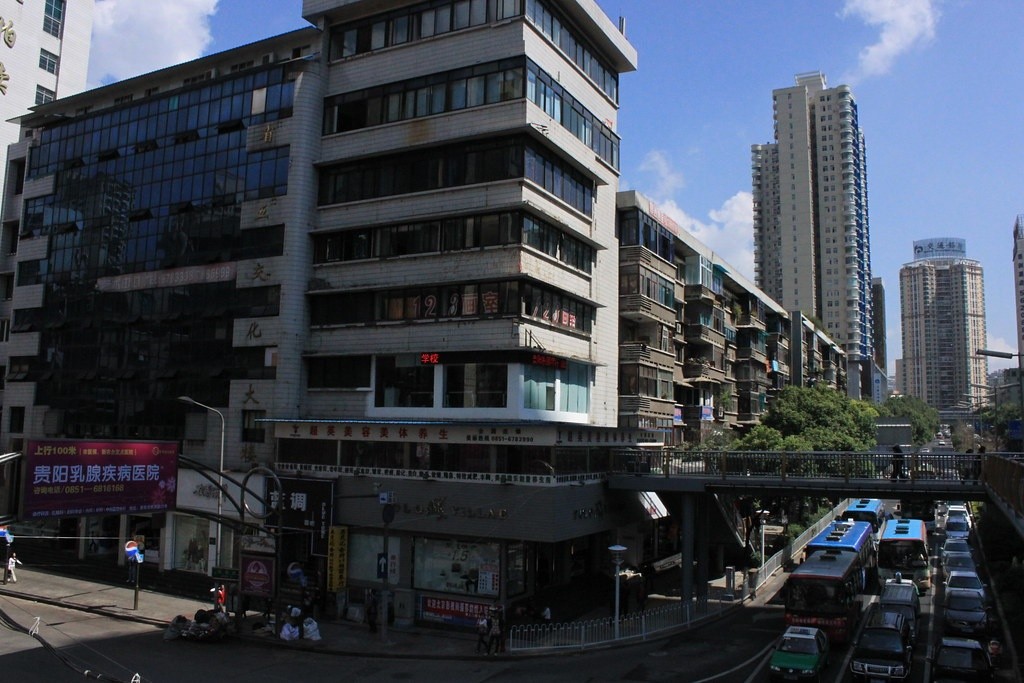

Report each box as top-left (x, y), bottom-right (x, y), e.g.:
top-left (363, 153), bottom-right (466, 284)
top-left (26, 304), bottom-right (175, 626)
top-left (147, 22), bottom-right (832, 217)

top-left (494, 653), bottom-right (498, 656)
top-left (132, 580), bottom-right (135, 583)
top-left (484, 652), bottom-right (490, 656)
top-left (127, 578), bottom-right (131, 582)
top-left (475, 649), bottom-right (479, 654)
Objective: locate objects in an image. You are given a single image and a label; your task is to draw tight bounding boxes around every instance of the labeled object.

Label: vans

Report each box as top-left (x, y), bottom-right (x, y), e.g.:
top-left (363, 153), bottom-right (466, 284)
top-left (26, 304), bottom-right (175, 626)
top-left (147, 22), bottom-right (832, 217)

top-left (874, 582), bottom-right (924, 640)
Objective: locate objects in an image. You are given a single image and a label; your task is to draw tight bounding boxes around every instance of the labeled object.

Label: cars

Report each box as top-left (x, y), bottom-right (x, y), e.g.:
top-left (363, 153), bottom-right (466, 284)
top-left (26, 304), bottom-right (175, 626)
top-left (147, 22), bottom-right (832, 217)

top-left (939, 502), bottom-right (980, 580)
top-left (935, 429), bottom-right (952, 439)
top-left (922, 448), bottom-right (930, 453)
top-left (924, 635), bottom-right (999, 683)
top-left (938, 440), bottom-right (946, 446)
top-left (941, 588), bottom-right (992, 639)
top-left (767, 627), bottom-right (830, 683)
top-left (848, 612), bottom-right (914, 683)
top-left (941, 569), bottom-right (989, 607)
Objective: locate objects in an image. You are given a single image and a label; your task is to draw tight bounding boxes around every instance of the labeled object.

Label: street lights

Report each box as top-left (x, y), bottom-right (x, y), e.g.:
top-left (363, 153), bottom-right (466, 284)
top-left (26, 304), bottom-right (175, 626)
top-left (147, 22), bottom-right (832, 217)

top-left (610, 544), bottom-right (626, 638)
top-left (970, 383), bottom-right (1021, 451)
top-left (176, 393), bottom-right (226, 613)
top-left (975, 348), bottom-right (1024, 445)
top-left (962, 393), bottom-right (998, 446)
top-left (757, 509), bottom-right (771, 570)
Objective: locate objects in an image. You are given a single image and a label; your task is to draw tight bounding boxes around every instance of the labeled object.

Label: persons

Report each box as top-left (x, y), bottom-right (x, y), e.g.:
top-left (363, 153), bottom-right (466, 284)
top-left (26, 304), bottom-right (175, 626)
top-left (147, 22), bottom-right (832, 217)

top-left (367, 599), bottom-right (378, 634)
top-left (473, 610), bottom-right (507, 657)
top-left (8, 552), bottom-right (23, 583)
top-left (286, 605), bottom-right (303, 638)
top-left (218, 585), bottom-right (225, 613)
top-left (889, 445), bottom-right (908, 482)
top-left (961, 449), bottom-right (974, 485)
top-left (971, 446), bottom-right (986, 486)
top-left (88, 531), bottom-right (99, 553)
top-left (540, 604), bottom-right (551, 632)
top-left (125, 561), bottom-right (137, 582)
top-left (263, 598), bottom-right (273, 617)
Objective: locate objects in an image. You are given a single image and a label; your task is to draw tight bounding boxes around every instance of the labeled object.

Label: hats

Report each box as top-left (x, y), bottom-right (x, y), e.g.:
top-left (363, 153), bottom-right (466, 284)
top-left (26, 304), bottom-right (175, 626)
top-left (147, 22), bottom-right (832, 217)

top-left (287, 605), bottom-right (291, 610)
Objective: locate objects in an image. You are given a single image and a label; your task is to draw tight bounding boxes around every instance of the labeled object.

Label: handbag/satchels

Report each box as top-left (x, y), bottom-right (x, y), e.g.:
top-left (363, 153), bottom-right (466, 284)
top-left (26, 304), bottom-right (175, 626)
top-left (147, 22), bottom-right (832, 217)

top-left (7, 569), bottom-right (12, 578)
top-left (476, 618), bottom-right (480, 631)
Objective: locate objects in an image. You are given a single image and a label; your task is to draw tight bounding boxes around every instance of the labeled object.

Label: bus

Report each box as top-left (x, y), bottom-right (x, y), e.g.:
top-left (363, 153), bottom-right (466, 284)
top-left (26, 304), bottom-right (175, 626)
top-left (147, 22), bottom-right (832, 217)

top-left (799, 520), bottom-right (875, 588)
top-left (900, 499), bottom-right (936, 531)
top-left (780, 547), bottom-right (863, 647)
top-left (875, 518), bottom-right (933, 595)
top-left (839, 497), bottom-right (885, 537)
